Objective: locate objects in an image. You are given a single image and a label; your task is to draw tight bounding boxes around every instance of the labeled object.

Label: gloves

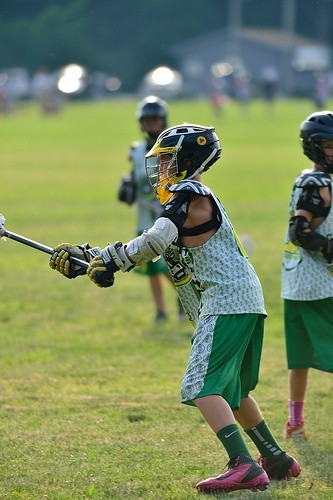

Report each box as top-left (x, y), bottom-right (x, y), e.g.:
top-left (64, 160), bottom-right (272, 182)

top-left (86, 241), bottom-right (134, 289)
top-left (117, 181), bottom-right (135, 203)
top-left (50, 240), bottom-right (101, 279)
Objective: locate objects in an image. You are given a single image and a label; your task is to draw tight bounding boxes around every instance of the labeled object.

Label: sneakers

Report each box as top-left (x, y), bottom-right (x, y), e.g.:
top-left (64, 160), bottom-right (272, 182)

top-left (256, 453), bottom-right (301, 481)
top-left (195, 455), bottom-right (270, 492)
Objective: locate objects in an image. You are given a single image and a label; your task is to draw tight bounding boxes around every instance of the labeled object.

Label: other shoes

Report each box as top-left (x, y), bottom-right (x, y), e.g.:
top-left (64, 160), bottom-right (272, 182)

top-left (154, 309), bottom-right (169, 324)
top-left (284, 419), bottom-right (306, 441)
top-left (178, 313), bottom-right (187, 323)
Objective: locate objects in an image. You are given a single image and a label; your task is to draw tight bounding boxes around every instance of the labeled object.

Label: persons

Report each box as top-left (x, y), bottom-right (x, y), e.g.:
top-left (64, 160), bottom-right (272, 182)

top-left (280, 110), bottom-right (333, 440)
top-left (50, 122), bottom-right (300, 491)
top-left (118, 96), bottom-right (188, 321)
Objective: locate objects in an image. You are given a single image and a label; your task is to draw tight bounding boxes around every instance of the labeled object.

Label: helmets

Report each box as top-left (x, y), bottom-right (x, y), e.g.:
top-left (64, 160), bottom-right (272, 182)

top-left (299, 110), bottom-right (333, 174)
top-left (136, 96), bottom-right (169, 122)
top-left (145, 123), bottom-right (222, 206)
